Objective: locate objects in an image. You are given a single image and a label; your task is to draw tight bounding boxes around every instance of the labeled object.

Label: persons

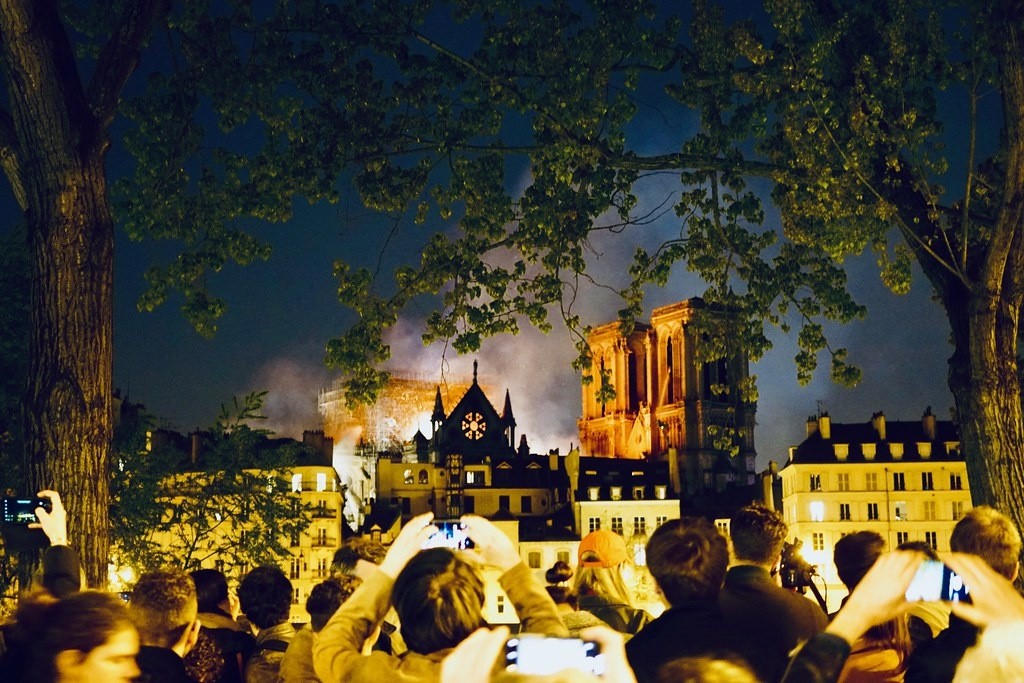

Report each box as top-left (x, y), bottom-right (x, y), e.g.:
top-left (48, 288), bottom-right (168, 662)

top-left (0, 490), bottom-right (81, 683)
top-left (827, 532), bottom-right (901, 650)
top-left (905, 507), bottom-right (1022, 683)
top-left (783, 550), bottom-right (1024, 683)
top-left (625, 517), bottom-right (787, 683)
top-left (184, 569), bottom-right (254, 683)
top-left (440, 625), bottom-right (638, 683)
top-left (895, 542), bottom-right (977, 683)
top-left (237, 564), bottom-right (296, 682)
top-left (331, 540), bottom-right (388, 593)
top-left (280, 579), bottom-right (351, 683)
top-left (30, 588), bottom-right (146, 683)
top-left (313, 513), bottom-right (570, 683)
top-left (128, 568), bottom-right (201, 682)
top-left (717, 504), bottom-right (831, 682)
top-left (567, 530), bottom-right (655, 635)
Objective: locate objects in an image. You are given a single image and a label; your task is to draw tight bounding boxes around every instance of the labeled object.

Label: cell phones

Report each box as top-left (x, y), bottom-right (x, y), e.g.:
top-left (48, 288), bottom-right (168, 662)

top-left (505, 637), bottom-right (603, 675)
top-left (3, 496), bottom-right (52, 523)
top-left (420, 520), bottom-right (474, 550)
top-left (905, 560), bottom-right (973, 606)
